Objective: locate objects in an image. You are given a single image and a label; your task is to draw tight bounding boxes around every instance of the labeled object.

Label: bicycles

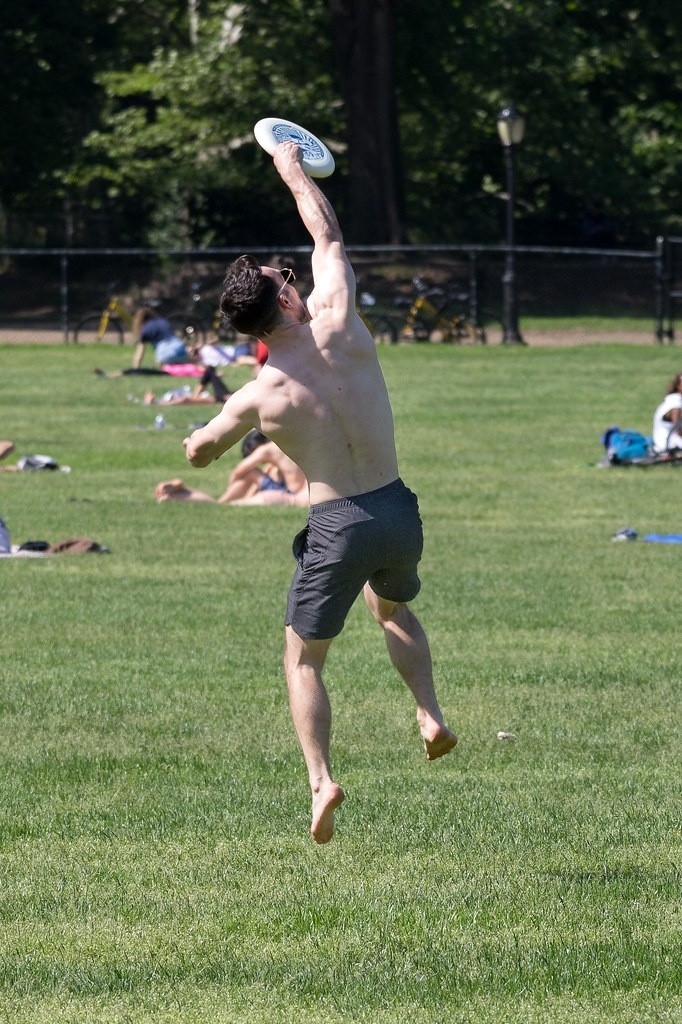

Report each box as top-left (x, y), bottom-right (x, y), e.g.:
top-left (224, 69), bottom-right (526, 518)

top-left (71, 255), bottom-right (502, 346)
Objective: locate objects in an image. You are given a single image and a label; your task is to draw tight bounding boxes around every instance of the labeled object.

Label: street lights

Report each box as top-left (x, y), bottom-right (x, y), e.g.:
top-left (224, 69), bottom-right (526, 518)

top-left (495, 101), bottom-right (527, 345)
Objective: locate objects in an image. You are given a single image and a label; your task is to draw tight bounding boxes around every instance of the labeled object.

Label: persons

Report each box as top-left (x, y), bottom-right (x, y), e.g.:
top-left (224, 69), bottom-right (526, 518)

top-left (97, 286), bottom-right (269, 403)
top-left (652, 370), bottom-right (682, 461)
top-left (217, 428), bottom-right (306, 504)
top-left (181, 141), bottom-right (458, 844)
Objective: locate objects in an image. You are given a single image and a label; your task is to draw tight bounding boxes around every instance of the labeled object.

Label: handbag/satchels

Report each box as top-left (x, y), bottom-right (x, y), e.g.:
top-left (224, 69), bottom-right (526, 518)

top-left (603, 426), bottom-right (655, 464)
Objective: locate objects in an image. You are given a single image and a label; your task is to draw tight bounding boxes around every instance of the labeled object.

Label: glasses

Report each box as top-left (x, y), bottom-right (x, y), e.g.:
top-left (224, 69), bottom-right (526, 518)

top-left (276, 268), bottom-right (296, 298)
